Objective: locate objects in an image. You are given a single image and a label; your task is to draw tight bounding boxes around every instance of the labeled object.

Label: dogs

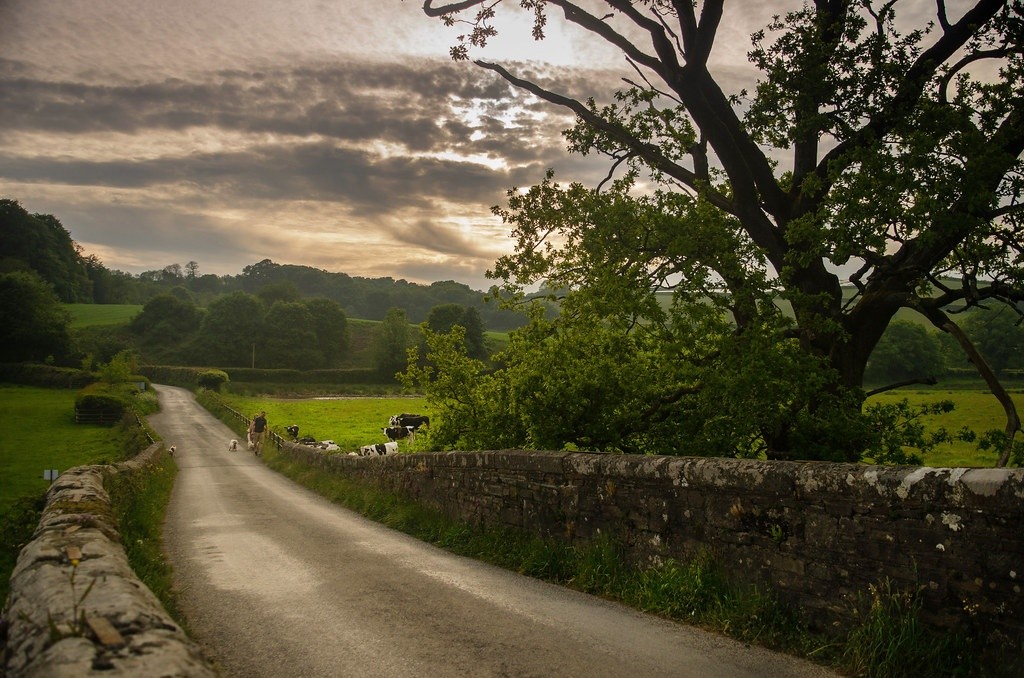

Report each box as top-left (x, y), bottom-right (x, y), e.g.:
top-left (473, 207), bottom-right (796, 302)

top-left (228, 439), bottom-right (239, 451)
top-left (169, 440), bottom-right (177, 458)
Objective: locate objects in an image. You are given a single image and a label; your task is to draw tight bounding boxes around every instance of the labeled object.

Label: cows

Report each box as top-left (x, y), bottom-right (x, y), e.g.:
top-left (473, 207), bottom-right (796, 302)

top-left (360, 413), bottom-right (430, 457)
top-left (285, 424), bottom-right (358, 456)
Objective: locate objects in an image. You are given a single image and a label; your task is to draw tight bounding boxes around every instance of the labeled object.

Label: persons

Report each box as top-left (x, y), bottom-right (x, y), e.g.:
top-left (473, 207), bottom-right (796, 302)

top-left (247, 413), bottom-right (259, 444)
top-left (251, 410), bottom-right (267, 456)
